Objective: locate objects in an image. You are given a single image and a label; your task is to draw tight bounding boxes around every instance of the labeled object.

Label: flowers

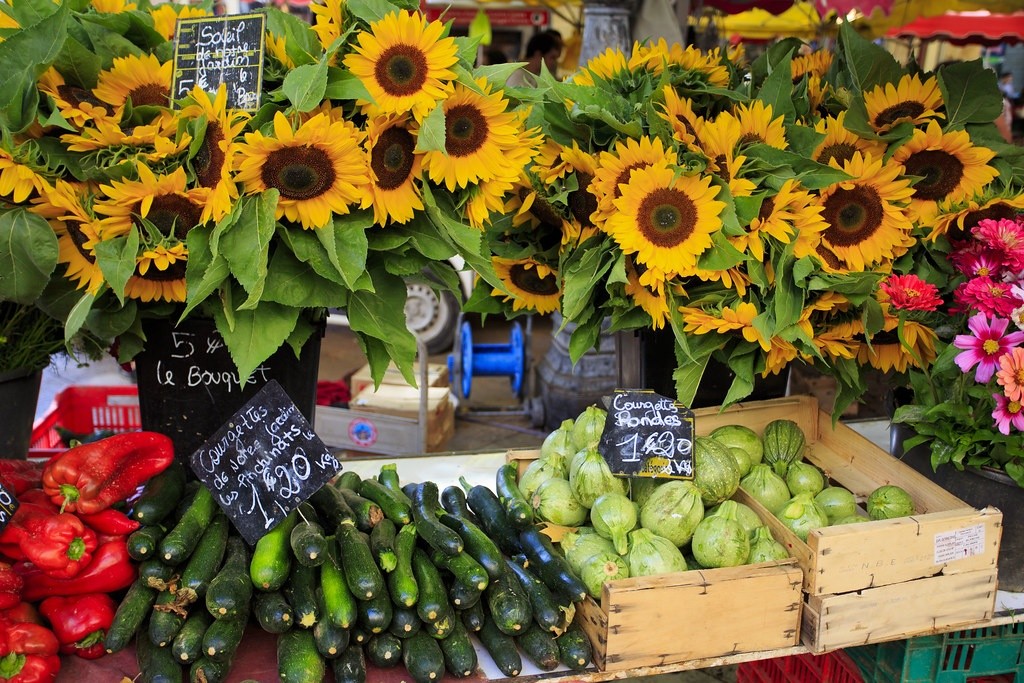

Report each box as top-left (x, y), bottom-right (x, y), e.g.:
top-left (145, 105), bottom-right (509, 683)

top-left (459, 15), bottom-right (1024, 430)
top-left (879, 219), bottom-right (1024, 487)
top-left (0, 0), bottom-right (546, 395)
top-left (0, 301), bottom-right (115, 377)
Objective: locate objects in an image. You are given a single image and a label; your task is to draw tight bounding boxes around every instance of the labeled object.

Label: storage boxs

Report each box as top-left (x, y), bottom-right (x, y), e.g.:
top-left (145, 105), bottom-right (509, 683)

top-left (348, 383), bottom-right (450, 447)
top-left (842, 610), bottom-right (1024, 683)
top-left (28, 384), bottom-right (142, 458)
top-left (688, 395), bottom-right (1005, 655)
top-left (735, 628), bottom-right (1015, 683)
top-left (350, 359), bottom-right (449, 400)
top-left (504, 447), bottom-right (804, 672)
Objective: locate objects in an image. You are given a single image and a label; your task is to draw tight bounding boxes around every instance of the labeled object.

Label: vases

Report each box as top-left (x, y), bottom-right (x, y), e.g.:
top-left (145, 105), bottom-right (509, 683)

top-left (890, 387), bottom-right (963, 480)
top-left (634, 326), bottom-right (790, 409)
top-left (0, 354), bottom-right (50, 460)
top-left (135, 306), bottom-right (327, 481)
top-left (936, 459), bottom-right (1024, 593)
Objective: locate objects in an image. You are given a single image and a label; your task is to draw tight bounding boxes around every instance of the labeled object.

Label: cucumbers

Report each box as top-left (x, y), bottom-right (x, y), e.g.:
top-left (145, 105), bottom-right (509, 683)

top-left (103, 463), bottom-right (592, 683)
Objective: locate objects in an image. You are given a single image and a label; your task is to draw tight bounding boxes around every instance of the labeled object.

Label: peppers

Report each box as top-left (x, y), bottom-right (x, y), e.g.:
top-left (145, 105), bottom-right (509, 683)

top-left (0, 431), bottom-right (172, 683)
